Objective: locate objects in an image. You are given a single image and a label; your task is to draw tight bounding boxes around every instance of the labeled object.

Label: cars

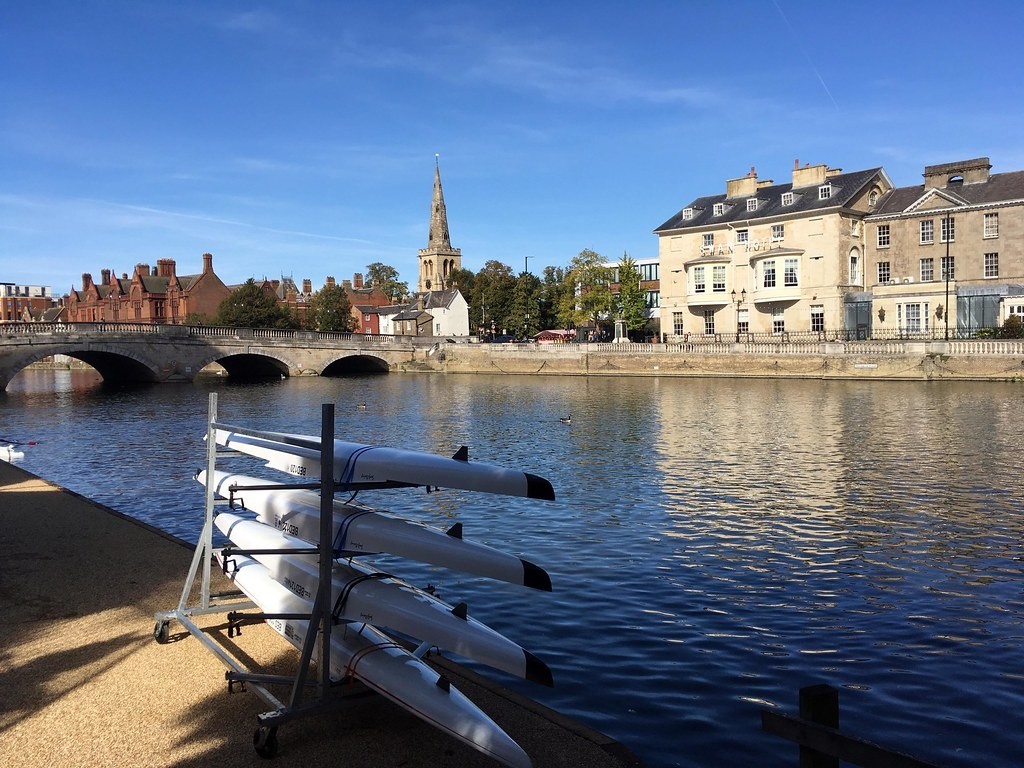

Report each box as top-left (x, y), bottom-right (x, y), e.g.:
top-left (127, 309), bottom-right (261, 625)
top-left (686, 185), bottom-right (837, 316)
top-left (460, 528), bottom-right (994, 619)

top-left (490, 336), bottom-right (517, 343)
top-left (523, 338), bottom-right (537, 343)
top-left (480, 336), bottom-right (491, 343)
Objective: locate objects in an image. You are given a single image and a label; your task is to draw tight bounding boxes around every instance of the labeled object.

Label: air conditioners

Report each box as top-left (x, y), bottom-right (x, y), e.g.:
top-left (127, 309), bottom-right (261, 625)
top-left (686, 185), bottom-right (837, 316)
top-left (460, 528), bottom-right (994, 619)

top-left (889, 277), bottom-right (900, 284)
top-left (903, 277), bottom-right (914, 283)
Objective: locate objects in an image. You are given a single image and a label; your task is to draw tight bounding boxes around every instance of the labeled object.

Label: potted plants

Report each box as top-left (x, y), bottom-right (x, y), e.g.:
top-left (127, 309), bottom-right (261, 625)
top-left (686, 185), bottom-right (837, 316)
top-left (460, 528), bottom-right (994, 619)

top-left (645, 322), bottom-right (660, 344)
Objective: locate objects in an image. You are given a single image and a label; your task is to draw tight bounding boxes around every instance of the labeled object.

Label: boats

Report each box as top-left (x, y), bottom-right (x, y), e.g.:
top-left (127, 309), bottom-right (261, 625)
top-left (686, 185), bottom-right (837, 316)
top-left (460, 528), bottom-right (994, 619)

top-left (191, 468), bottom-right (552, 594)
top-left (0, 445), bottom-right (24, 459)
top-left (214, 511), bottom-right (554, 690)
top-left (215, 548), bottom-right (532, 767)
top-left (203, 424), bottom-right (556, 503)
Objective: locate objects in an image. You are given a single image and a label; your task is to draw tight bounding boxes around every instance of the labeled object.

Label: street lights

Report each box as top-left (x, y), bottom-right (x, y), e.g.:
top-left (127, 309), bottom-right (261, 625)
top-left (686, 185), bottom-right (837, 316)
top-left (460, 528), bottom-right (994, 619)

top-left (731, 288), bottom-right (747, 343)
top-left (524, 256), bottom-right (534, 343)
